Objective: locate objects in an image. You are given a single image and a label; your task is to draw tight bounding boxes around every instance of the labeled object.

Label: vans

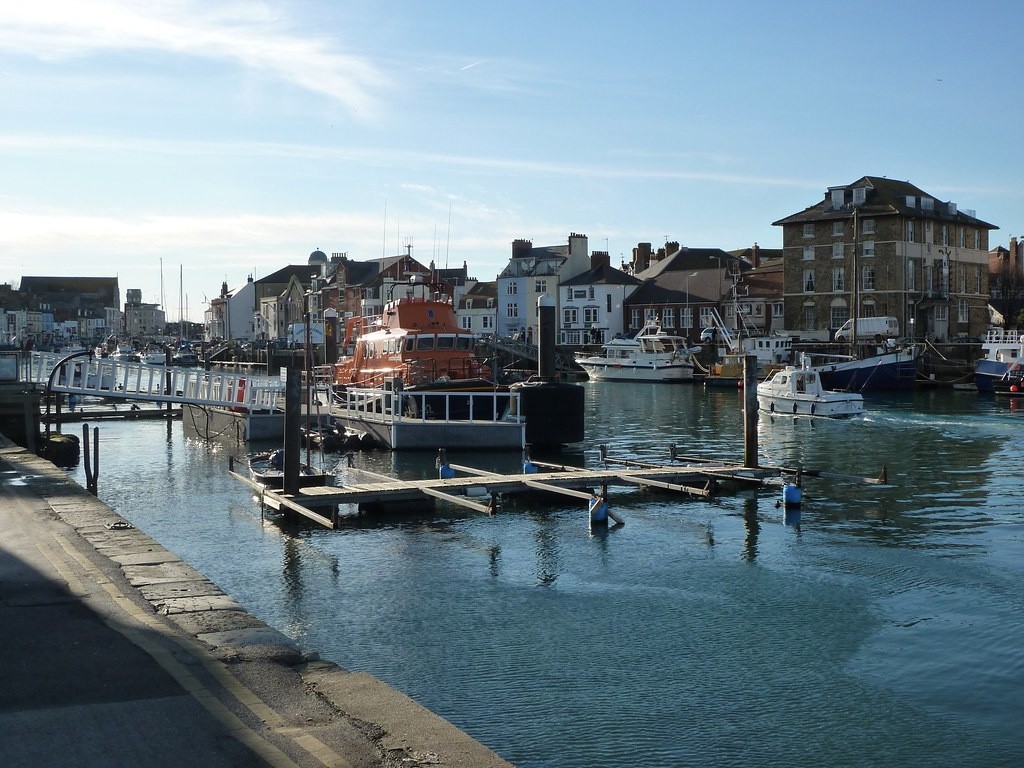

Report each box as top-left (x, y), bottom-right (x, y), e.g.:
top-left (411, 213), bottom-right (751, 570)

top-left (835, 317), bottom-right (900, 344)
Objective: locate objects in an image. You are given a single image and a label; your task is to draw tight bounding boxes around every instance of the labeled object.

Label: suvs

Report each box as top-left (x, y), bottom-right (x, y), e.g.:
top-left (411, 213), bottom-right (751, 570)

top-left (701, 327), bottom-right (736, 345)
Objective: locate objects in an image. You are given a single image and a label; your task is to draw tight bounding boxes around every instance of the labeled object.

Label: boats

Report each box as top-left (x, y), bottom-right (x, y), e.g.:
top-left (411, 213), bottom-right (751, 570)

top-left (19, 359), bottom-right (116, 390)
top-left (757, 351), bottom-right (862, 417)
top-left (704, 260), bottom-right (919, 393)
top-left (93, 333), bottom-right (199, 367)
top-left (974, 331), bottom-right (1024, 394)
top-left (573, 319), bottom-right (696, 382)
top-left (66, 344), bottom-right (87, 352)
top-left (303, 421), bottom-right (375, 452)
top-left (308, 274), bottom-right (515, 415)
